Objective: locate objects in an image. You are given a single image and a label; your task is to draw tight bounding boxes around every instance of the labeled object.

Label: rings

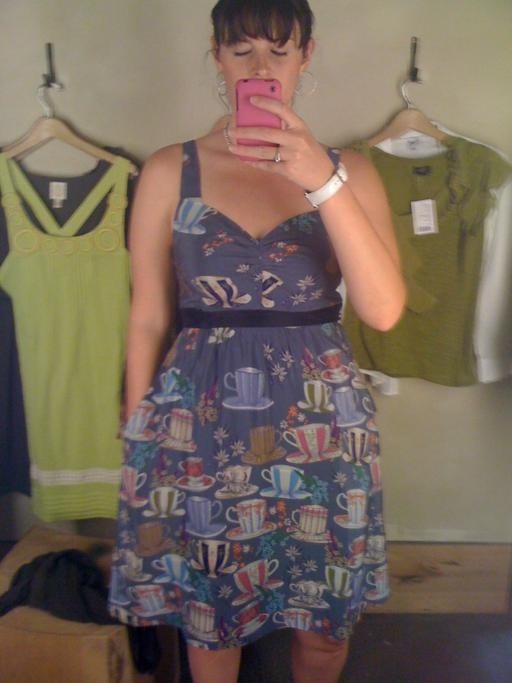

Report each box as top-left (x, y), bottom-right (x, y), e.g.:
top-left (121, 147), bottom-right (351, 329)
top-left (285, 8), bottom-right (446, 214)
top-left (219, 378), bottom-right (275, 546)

top-left (274, 146), bottom-right (281, 163)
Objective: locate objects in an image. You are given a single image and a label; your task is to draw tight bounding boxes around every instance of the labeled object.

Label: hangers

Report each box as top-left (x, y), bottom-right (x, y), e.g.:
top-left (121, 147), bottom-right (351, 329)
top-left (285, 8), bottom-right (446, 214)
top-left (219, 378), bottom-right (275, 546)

top-left (349, 76), bottom-right (457, 148)
top-left (0, 85), bottom-right (140, 177)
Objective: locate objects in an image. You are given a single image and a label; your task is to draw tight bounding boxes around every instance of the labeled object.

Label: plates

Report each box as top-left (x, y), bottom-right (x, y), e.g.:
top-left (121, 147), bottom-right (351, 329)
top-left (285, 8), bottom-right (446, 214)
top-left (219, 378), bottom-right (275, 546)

top-left (172, 223), bottom-right (208, 238)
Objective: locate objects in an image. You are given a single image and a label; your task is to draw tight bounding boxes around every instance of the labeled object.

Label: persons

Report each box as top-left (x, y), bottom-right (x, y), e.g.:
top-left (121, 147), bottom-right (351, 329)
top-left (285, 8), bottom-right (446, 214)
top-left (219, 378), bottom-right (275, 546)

top-left (106, 0), bottom-right (409, 681)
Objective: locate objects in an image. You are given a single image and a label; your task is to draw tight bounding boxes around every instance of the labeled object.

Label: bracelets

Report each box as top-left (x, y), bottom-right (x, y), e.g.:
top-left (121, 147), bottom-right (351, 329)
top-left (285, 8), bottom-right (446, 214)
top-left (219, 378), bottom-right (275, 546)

top-left (303, 161), bottom-right (348, 209)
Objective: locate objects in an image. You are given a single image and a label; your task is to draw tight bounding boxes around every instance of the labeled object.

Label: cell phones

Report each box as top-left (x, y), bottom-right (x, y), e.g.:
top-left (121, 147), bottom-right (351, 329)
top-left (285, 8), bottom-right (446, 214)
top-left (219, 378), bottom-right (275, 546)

top-left (235, 77), bottom-right (283, 162)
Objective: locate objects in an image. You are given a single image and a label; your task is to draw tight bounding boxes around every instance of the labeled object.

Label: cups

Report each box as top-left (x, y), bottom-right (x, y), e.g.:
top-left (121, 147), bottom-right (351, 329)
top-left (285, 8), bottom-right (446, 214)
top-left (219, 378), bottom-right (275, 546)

top-left (176, 195), bottom-right (217, 229)
top-left (109, 346), bottom-right (388, 643)
